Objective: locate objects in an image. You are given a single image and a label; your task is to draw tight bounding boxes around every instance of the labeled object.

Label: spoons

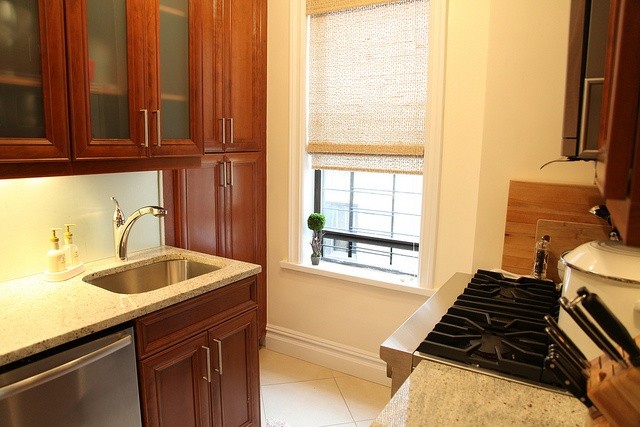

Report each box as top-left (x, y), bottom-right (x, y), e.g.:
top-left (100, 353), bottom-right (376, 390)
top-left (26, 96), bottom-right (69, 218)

top-left (587, 204), bottom-right (613, 228)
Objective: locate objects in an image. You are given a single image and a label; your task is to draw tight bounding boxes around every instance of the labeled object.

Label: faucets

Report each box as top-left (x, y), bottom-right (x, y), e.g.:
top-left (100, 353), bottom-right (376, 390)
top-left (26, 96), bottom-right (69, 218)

top-left (107, 196), bottom-right (166, 259)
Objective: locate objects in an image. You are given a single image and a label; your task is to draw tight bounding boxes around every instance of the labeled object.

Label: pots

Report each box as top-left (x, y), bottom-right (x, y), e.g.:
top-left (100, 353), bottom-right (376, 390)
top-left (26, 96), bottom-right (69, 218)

top-left (557, 238), bottom-right (640, 361)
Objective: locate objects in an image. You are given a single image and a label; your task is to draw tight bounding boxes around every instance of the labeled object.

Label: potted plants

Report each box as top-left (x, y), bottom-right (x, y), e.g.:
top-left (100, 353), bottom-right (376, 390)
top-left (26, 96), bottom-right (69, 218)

top-left (308, 213), bottom-right (326, 265)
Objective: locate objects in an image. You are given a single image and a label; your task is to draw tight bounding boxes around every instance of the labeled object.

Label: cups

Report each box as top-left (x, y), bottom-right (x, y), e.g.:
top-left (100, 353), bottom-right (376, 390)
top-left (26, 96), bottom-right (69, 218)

top-left (533, 240), bottom-right (551, 280)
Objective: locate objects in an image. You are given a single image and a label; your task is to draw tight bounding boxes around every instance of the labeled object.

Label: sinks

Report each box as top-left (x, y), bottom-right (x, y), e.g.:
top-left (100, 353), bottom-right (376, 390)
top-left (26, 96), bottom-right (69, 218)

top-left (81, 255), bottom-right (228, 297)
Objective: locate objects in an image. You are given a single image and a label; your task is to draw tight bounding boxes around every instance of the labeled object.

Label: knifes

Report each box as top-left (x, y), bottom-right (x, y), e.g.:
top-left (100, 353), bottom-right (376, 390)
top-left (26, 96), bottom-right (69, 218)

top-left (542, 286), bottom-right (640, 421)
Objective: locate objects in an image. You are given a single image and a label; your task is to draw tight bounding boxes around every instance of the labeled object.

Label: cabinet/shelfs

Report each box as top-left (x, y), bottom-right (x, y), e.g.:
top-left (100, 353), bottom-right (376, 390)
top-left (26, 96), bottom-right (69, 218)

top-left (561, 1), bottom-right (640, 245)
top-left (163, 152), bottom-right (267, 331)
top-left (204, 0), bottom-right (268, 154)
top-left (134, 274), bottom-right (261, 426)
top-left (66, 0), bottom-right (204, 160)
top-left (0, 0), bottom-right (72, 164)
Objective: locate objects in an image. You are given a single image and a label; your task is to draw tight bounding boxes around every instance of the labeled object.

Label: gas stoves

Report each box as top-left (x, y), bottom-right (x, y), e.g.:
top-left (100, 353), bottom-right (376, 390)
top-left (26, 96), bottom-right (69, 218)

top-left (418, 268), bottom-right (569, 393)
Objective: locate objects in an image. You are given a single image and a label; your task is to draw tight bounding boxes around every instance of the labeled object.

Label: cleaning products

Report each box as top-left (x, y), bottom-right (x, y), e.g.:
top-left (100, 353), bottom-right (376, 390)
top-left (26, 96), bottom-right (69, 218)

top-left (47, 227), bottom-right (65, 273)
top-left (60, 224), bottom-right (79, 266)
